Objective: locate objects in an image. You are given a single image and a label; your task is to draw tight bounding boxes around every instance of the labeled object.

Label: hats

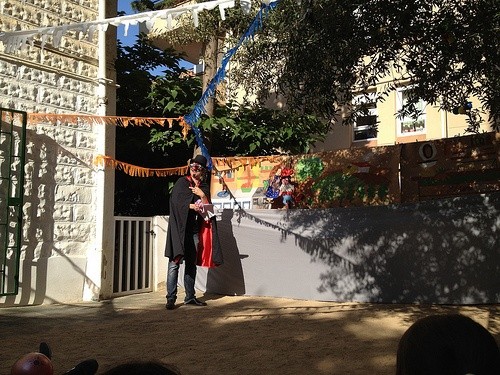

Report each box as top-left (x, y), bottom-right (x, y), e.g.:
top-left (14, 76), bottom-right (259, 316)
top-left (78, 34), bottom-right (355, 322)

top-left (190, 155), bottom-right (208, 170)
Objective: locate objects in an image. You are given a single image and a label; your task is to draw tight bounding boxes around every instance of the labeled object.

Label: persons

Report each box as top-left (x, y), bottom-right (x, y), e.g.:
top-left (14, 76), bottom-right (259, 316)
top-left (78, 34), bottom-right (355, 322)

top-left (163, 154), bottom-right (225, 311)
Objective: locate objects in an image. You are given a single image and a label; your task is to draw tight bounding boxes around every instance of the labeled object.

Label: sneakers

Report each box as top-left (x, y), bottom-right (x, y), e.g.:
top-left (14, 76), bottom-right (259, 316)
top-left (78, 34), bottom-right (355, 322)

top-left (63, 358), bottom-right (98, 375)
top-left (39, 342), bottom-right (52, 361)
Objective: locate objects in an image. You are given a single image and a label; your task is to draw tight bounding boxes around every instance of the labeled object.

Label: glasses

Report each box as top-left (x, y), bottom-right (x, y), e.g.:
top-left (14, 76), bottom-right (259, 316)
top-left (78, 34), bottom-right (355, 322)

top-left (190, 164), bottom-right (207, 170)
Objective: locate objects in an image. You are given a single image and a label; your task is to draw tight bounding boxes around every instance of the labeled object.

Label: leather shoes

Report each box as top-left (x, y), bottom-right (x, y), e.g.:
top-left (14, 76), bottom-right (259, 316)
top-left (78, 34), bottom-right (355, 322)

top-left (184, 298), bottom-right (207, 306)
top-left (166, 300), bottom-right (176, 309)
